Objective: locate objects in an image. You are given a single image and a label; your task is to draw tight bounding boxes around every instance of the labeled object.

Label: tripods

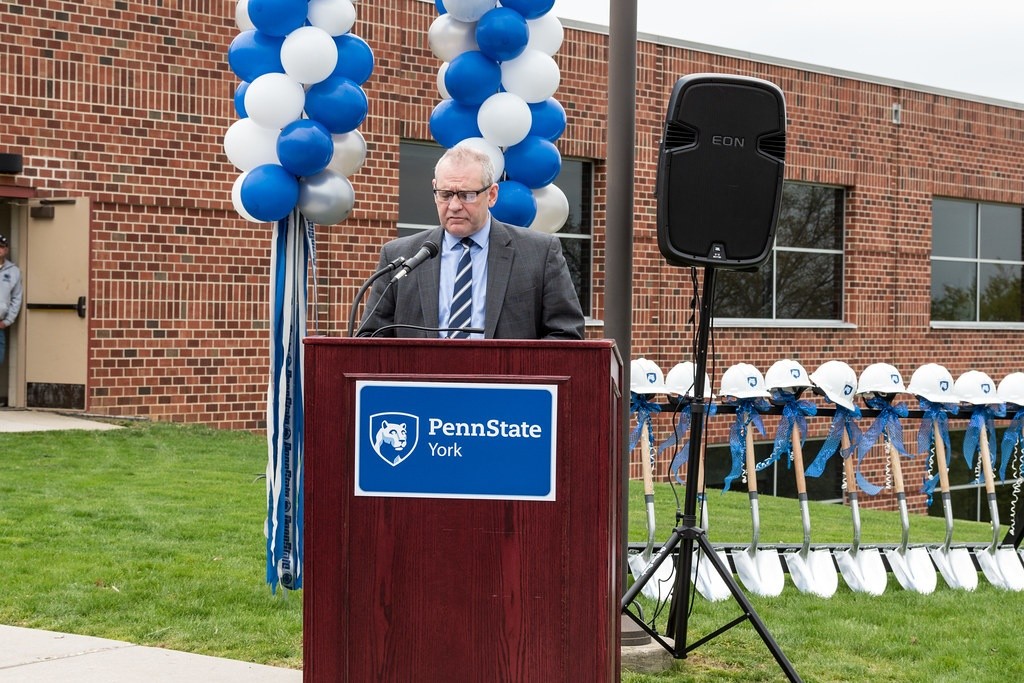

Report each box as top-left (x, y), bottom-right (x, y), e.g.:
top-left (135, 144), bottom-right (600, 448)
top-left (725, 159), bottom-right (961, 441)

top-left (620, 266), bottom-right (803, 683)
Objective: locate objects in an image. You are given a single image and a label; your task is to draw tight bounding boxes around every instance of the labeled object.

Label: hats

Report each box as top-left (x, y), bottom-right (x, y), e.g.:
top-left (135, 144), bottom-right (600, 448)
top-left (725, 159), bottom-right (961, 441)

top-left (0, 235), bottom-right (8, 244)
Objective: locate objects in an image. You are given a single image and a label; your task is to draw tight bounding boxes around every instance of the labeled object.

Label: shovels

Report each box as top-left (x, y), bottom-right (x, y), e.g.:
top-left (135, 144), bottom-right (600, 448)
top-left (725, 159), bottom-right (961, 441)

top-left (730, 397), bottom-right (786, 599)
top-left (778, 386), bottom-right (840, 599)
top-left (831, 396), bottom-right (889, 598)
top-left (972, 401), bottom-right (1024, 596)
top-left (925, 402), bottom-right (979, 594)
top-left (873, 392), bottom-right (939, 597)
top-left (690, 395), bottom-right (735, 603)
top-left (628, 395), bottom-right (679, 604)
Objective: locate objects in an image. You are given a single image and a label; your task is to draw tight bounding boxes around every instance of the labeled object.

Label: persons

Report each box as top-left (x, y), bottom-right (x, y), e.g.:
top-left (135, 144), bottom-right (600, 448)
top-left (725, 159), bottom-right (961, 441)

top-left (356, 144), bottom-right (587, 340)
top-left (0, 234), bottom-right (23, 407)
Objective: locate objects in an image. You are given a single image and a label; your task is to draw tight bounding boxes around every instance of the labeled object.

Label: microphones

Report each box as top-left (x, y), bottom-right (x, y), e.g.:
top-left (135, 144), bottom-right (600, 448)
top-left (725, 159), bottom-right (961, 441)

top-left (371, 324), bottom-right (485, 338)
top-left (391, 240), bottom-right (439, 284)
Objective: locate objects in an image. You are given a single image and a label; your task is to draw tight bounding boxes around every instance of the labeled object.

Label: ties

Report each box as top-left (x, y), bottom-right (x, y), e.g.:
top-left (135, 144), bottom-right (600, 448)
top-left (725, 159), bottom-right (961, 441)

top-left (447, 237), bottom-right (478, 338)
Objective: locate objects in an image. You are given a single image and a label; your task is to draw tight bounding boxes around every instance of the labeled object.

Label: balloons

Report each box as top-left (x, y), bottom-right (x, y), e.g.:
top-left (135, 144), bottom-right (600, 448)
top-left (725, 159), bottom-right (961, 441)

top-left (221, 0), bottom-right (378, 227)
top-left (425, 0), bottom-right (570, 233)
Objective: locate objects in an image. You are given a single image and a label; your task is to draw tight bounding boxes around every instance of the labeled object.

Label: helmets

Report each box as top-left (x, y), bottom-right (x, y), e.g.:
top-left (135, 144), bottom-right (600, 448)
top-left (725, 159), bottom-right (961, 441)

top-left (907, 363), bottom-right (960, 402)
top-left (997, 372), bottom-right (1024, 406)
top-left (856, 363), bottom-right (912, 394)
top-left (954, 370), bottom-right (1004, 404)
top-left (630, 358), bottom-right (670, 394)
top-left (765, 359), bottom-right (815, 390)
top-left (666, 362), bottom-right (717, 398)
top-left (720, 363), bottom-right (771, 398)
top-left (809, 361), bottom-right (857, 411)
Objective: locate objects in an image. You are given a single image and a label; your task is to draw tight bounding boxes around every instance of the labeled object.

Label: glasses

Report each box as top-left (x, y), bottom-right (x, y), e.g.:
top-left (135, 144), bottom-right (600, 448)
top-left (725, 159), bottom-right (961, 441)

top-left (0, 243), bottom-right (6, 248)
top-left (432, 183), bottom-right (493, 204)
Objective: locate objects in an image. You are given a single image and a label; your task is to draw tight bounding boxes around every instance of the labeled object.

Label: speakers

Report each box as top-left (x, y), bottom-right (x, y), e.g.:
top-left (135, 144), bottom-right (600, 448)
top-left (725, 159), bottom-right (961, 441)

top-left (657, 72), bottom-right (787, 267)
